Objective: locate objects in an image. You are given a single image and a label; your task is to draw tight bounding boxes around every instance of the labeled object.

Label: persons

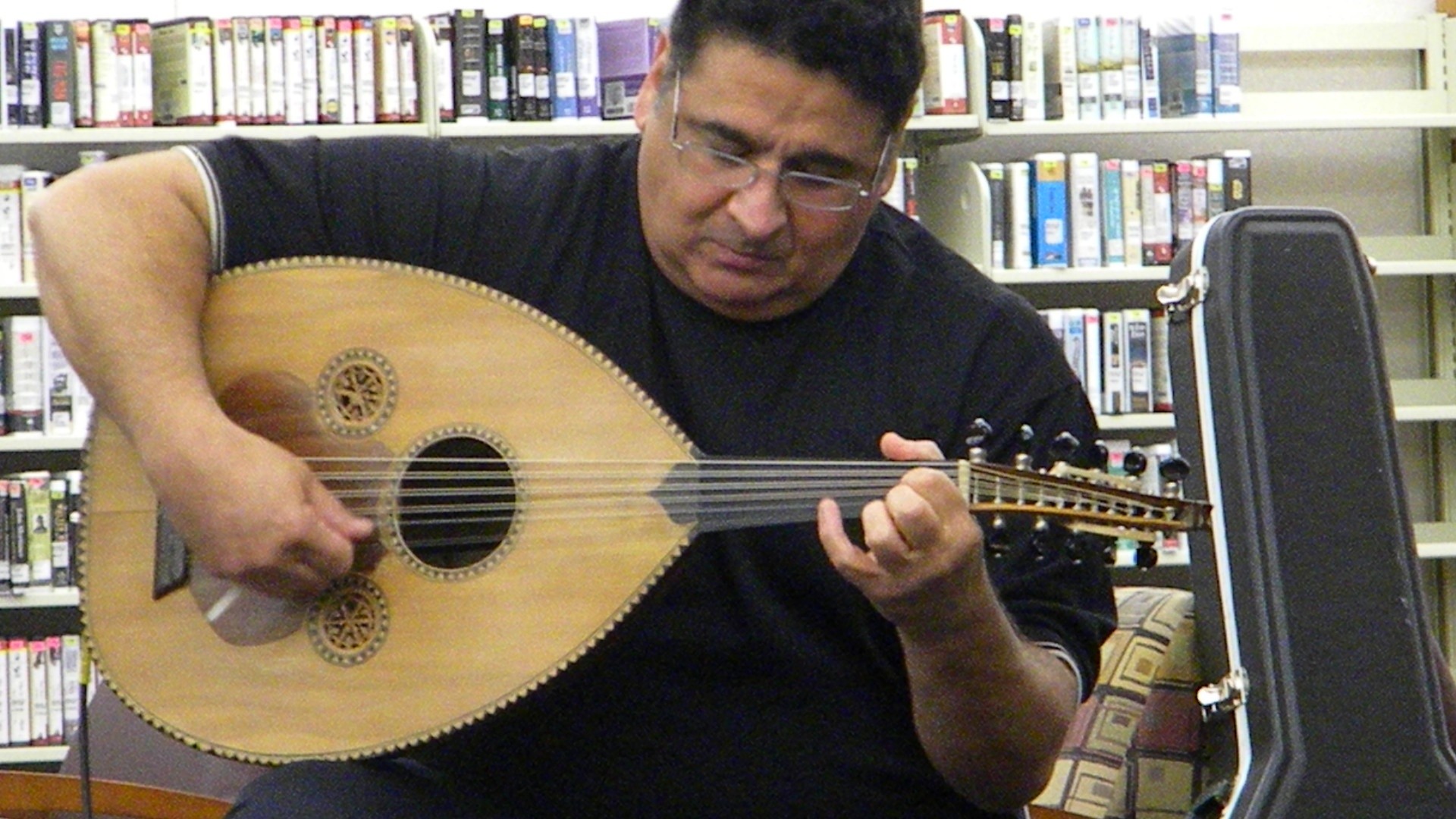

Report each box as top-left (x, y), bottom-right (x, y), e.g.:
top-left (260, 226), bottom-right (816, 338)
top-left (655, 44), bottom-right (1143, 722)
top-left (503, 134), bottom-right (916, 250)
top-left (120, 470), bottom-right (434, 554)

top-left (25, 0), bottom-right (1118, 819)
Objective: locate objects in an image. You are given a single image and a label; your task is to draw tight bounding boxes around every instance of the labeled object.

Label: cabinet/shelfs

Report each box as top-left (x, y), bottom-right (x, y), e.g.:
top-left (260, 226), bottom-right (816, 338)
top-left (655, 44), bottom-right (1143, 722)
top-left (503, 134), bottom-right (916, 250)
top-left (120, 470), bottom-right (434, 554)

top-left (0, 15), bottom-right (1456, 761)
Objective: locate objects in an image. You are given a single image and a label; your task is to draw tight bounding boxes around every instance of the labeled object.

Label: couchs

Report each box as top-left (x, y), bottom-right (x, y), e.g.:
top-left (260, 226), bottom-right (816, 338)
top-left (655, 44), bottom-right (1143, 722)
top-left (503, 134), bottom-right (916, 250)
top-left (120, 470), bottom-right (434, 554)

top-left (1018, 589), bottom-right (1201, 819)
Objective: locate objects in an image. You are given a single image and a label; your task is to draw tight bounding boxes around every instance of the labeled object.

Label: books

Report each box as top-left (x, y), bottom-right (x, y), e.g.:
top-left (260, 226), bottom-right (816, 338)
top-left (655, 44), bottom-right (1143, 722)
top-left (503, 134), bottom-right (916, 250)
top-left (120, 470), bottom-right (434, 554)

top-left (1099, 438), bottom-right (1190, 566)
top-left (1035, 307), bottom-right (1174, 417)
top-left (0, 8), bottom-right (1247, 127)
top-left (0, 151), bottom-right (106, 287)
top-left (0, 314), bottom-right (94, 437)
top-left (0, 470), bottom-right (84, 596)
top-left (878, 148), bottom-right (1254, 271)
top-left (0, 633), bottom-right (103, 747)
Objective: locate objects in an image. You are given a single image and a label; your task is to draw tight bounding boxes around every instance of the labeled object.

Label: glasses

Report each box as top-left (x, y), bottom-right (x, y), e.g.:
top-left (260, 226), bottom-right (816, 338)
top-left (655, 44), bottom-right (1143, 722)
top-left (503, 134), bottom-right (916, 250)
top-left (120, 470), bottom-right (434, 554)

top-left (667, 57), bottom-right (896, 213)
